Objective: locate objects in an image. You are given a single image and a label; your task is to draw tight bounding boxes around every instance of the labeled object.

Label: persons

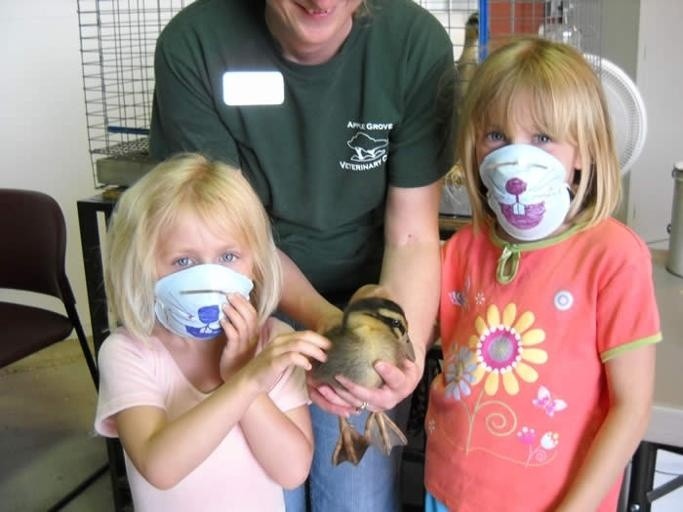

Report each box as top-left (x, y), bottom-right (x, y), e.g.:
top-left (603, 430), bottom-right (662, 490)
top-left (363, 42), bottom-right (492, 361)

top-left (94, 151), bottom-right (318, 512)
top-left (417, 25), bottom-right (666, 511)
top-left (148, 0), bottom-right (464, 510)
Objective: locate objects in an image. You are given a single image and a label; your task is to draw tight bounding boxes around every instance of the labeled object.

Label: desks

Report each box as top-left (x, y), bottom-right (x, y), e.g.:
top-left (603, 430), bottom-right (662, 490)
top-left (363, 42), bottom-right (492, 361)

top-left (619, 238), bottom-right (683, 512)
top-left (76, 185), bottom-right (471, 368)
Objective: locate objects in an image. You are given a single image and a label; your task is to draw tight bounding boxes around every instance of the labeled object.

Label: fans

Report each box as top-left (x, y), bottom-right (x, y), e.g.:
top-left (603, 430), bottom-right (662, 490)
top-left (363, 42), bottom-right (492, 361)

top-left (581, 53), bottom-right (648, 179)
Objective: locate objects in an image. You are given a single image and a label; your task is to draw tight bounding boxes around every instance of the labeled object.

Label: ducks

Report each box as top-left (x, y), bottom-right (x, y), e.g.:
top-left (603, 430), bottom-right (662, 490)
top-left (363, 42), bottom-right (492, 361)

top-left (306, 284), bottom-right (416, 467)
top-left (454, 13), bottom-right (480, 102)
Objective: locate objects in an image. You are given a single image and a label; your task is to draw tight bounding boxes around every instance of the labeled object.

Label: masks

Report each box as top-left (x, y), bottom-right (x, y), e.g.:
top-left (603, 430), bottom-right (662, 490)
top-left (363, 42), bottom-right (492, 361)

top-left (152, 265), bottom-right (253, 340)
top-left (479, 146), bottom-right (575, 240)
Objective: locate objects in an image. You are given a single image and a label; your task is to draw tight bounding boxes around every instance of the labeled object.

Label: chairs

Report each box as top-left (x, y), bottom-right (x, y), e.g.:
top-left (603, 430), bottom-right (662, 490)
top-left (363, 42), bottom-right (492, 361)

top-left (0, 188), bottom-right (127, 512)
